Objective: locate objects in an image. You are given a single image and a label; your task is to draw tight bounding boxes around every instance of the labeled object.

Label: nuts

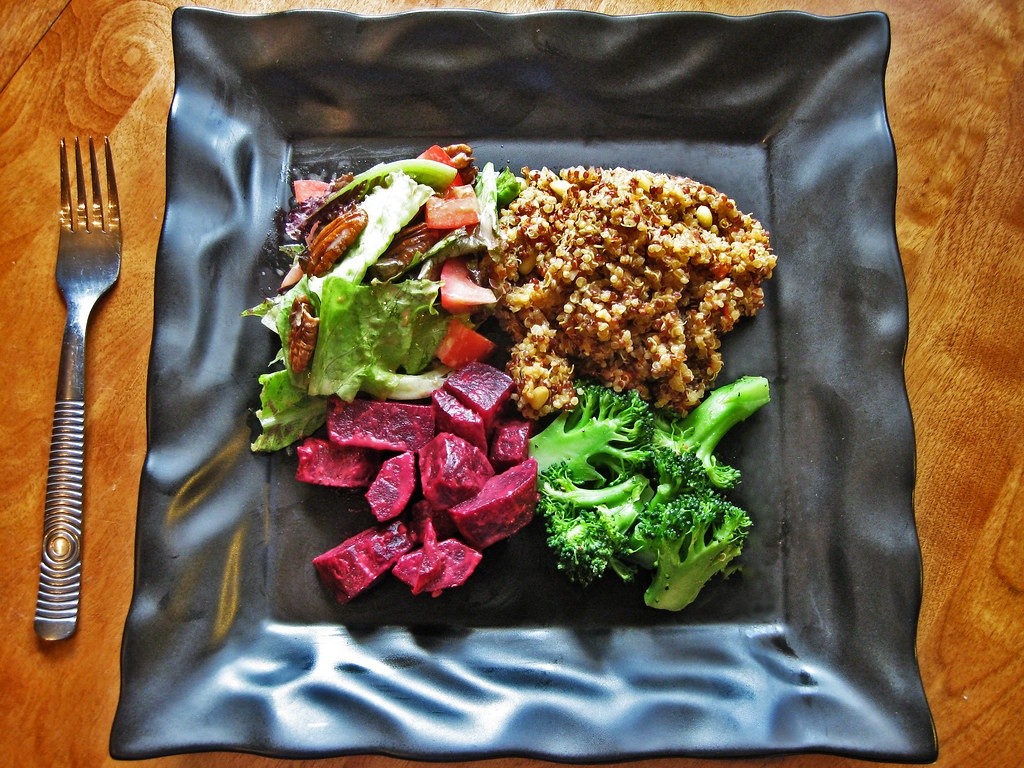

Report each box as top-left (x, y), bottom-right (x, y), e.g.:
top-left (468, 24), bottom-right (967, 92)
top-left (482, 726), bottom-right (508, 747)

top-left (442, 143), bottom-right (479, 184)
top-left (288, 295), bottom-right (320, 374)
top-left (298, 207), bottom-right (368, 277)
top-left (382, 219), bottom-right (436, 268)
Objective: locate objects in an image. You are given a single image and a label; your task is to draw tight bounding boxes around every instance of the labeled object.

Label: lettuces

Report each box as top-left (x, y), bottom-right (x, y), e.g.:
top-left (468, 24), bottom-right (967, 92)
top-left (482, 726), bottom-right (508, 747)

top-left (243, 159), bottom-right (523, 452)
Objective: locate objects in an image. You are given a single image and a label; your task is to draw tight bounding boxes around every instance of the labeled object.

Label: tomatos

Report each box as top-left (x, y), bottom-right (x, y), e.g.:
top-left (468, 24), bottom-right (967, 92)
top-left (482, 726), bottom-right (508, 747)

top-left (414, 146), bottom-right (498, 368)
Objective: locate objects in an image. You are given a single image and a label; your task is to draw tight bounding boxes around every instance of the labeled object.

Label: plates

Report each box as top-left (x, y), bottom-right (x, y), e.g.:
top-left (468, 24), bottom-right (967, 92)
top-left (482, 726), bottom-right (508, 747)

top-left (109, 6), bottom-right (940, 763)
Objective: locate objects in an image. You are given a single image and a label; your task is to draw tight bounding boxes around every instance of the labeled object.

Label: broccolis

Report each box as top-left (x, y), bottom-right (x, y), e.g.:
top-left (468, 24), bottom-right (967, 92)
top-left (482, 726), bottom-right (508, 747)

top-left (522, 375), bottom-right (770, 612)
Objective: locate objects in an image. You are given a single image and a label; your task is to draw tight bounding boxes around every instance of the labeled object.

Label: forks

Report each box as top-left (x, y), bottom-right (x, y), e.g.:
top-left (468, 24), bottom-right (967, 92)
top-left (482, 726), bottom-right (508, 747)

top-left (35, 135), bottom-right (123, 641)
